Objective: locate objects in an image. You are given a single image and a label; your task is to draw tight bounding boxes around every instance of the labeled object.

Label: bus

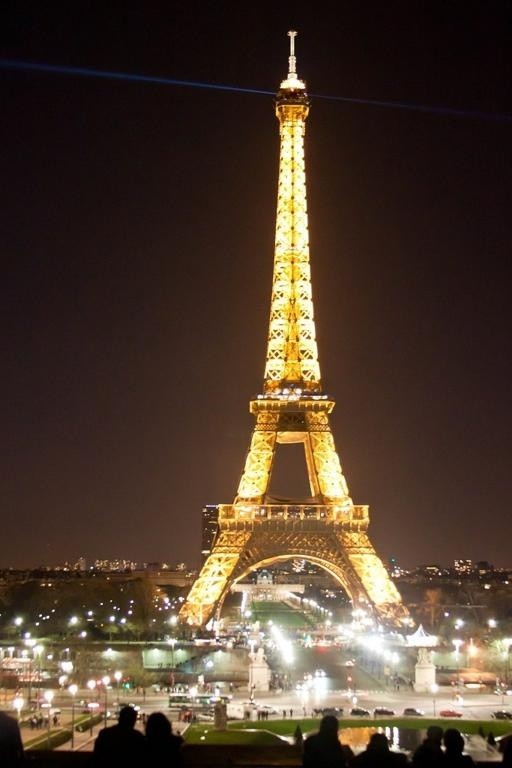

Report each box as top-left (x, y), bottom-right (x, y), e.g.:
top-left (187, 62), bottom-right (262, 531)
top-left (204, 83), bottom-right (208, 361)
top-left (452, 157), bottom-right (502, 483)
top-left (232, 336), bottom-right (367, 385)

top-left (169, 693), bottom-right (230, 710)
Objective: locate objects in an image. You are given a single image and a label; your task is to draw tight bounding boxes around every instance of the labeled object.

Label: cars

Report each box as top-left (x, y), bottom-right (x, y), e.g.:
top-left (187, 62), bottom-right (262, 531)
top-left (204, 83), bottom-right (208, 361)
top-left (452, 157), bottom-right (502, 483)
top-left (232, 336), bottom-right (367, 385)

top-left (349, 708), bottom-right (369, 716)
top-left (491, 711), bottom-right (512, 720)
top-left (296, 669), bottom-right (326, 690)
top-left (439, 710), bottom-right (462, 717)
top-left (256, 704), bottom-right (278, 714)
top-left (321, 706), bottom-right (344, 717)
top-left (374, 707), bottom-right (394, 716)
top-left (404, 708), bottom-right (424, 716)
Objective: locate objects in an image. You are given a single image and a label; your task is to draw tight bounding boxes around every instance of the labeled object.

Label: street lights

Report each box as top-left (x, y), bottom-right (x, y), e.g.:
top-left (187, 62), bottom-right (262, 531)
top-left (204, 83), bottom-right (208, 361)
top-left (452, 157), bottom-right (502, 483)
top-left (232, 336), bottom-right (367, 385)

top-left (45, 690), bottom-right (55, 751)
top-left (14, 698), bottom-right (24, 719)
top-left (87, 679), bottom-right (97, 737)
top-left (102, 675), bottom-right (111, 729)
top-left (114, 671), bottom-right (123, 720)
top-left (68, 684), bottom-right (78, 748)
top-left (167, 639), bottom-right (178, 687)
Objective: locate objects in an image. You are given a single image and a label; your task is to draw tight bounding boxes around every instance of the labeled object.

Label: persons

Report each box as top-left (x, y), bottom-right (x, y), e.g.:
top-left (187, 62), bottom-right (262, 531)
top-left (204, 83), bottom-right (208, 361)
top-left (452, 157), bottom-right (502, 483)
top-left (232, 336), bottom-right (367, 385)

top-left (144, 710), bottom-right (186, 767)
top-left (160, 682), bottom-right (235, 697)
top-left (29, 712), bottom-right (63, 731)
top-left (410, 726), bottom-right (445, 767)
top-left (93, 705), bottom-right (146, 767)
top-left (352, 733), bottom-right (411, 768)
top-left (256, 706), bottom-right (344, 720)
top-left (441, 727), bottom-right (474, 768)
top-left (304, 713), bottom-right (357, 767)
